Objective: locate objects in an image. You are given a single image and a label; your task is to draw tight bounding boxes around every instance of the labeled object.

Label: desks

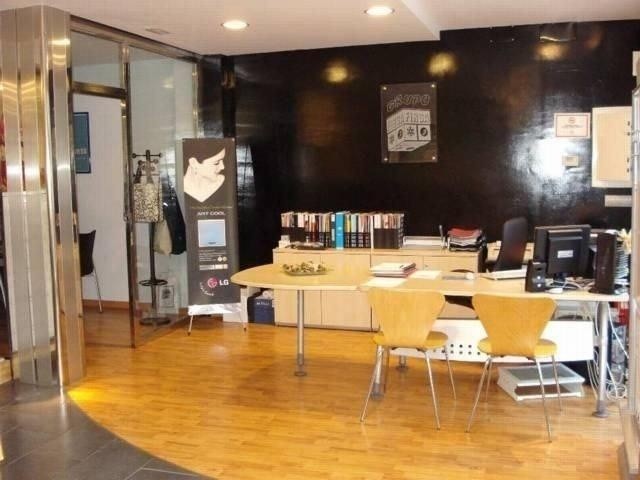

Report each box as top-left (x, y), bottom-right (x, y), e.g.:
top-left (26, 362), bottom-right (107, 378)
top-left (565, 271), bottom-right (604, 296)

top-left (229, 262), bottom-right (630, 418)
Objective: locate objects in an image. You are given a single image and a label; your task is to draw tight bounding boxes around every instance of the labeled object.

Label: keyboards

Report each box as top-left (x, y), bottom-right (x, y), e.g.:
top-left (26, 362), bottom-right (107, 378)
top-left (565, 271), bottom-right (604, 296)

top-left (481, 269), bottom-right (526, 280)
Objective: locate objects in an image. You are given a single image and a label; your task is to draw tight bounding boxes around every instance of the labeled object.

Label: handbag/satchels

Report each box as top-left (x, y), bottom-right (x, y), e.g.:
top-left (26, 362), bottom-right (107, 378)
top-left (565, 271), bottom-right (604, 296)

top-left (133, 182), bottom-right (163, 224)
top-left (154, 218), bottom-right (172, 255)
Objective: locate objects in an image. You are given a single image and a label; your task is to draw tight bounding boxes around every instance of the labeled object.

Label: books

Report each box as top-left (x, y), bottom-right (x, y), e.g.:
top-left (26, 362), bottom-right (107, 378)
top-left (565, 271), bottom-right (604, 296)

top-left (279, 211), bottom-right (406, 249)
top-left (368, 259), bottom-right (417, 279)
top-left (447, 226), bottom-right (485, 253)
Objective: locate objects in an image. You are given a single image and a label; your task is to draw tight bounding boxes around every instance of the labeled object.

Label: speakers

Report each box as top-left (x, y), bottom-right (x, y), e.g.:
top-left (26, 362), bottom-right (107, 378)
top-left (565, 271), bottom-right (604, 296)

top-left (526, 259), bottom-right (547, 292)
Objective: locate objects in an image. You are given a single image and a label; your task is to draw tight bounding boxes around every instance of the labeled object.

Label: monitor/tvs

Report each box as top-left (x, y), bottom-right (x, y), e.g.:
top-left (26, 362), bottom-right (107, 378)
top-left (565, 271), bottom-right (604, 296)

top-left (533, 224), bottom-right (591, 289)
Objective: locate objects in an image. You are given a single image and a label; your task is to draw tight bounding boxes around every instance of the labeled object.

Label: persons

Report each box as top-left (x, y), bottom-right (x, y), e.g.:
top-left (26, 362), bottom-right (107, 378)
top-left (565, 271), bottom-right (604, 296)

top-left (183, 138), bottom-right (226, 206)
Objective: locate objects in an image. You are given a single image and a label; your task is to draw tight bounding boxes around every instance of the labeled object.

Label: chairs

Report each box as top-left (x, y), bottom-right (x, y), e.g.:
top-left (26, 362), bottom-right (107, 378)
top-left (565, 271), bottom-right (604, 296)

top-left (489, 217), bottom-right (529, 270)
top-left (80, 229), bottom-right (103, 313)
top-left (360, 286), bottom-right (565, 444)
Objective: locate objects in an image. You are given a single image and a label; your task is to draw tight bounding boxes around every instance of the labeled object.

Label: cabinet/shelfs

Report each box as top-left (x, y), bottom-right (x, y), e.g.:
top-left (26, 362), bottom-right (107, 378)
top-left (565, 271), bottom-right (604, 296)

top-left (271, 245), bottom-right (482, 333)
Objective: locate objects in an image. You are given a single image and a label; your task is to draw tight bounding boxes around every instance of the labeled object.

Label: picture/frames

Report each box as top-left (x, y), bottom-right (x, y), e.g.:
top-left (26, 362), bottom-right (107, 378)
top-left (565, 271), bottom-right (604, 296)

top-left (591, 106), bottom-right (633, 188)
top-left (74, 112), bottom-right (91, 173)
